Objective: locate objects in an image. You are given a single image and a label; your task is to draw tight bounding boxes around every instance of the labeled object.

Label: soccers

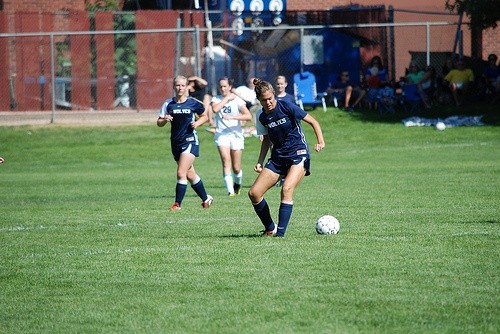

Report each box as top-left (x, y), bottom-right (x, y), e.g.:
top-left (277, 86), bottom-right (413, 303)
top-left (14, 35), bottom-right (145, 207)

top-left (316, 215), bottom-right (340, 235)
top-left (436, 122), bottom-right (446, 130)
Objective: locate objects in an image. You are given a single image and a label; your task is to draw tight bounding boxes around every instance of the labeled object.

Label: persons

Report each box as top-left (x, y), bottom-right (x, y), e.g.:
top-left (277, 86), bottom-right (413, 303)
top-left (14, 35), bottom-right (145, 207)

top-left (327, 47), bottom-right (500, 112)
top-left (185, 75), bottom-right (301, 186)
top-left (248, 79), bottom-right (326, 236)
top-left (210, 77), bottom-right (252, 197)
top-left (156, 75), bottom-right (213, 212)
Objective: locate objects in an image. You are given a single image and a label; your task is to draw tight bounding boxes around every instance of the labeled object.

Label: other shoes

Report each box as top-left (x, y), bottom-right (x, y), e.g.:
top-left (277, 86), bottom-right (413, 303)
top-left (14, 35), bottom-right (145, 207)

top-left (341, 106), bottom-right (355, 112)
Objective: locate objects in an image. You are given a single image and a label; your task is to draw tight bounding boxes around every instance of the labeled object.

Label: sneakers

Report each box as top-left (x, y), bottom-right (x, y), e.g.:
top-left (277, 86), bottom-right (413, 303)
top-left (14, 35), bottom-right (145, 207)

top-left (228, 182), bottom-right (241, 197)
top-left (263, 224), bottom-right (279, 237)
top-left (201, 195), bottom-right (213, 208)
top-left (169, 202), bottom-right (182, 210)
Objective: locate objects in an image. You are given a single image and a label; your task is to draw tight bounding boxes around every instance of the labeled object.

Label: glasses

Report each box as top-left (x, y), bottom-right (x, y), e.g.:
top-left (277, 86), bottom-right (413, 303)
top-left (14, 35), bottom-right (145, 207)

top-left (488, 58), bottom-right (494, 61)
top-left (341, 74), bottom-right (349, 77)
top-left (457, 64), bottom-right (464, 66)
top-left (372, 63), bottom-right (378, 65)
top-left (411, 66), bottom-right (416, 68)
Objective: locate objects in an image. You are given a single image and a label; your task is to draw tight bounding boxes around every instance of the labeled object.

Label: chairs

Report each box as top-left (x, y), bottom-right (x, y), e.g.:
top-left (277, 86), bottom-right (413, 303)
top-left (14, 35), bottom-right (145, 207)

top-left (325, 73), bottom-right (345, 109)
top-left (293, 72), bottom-right (328, 114)
